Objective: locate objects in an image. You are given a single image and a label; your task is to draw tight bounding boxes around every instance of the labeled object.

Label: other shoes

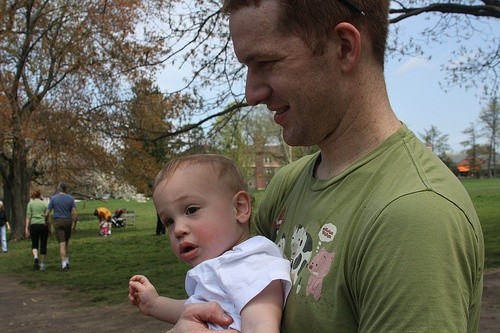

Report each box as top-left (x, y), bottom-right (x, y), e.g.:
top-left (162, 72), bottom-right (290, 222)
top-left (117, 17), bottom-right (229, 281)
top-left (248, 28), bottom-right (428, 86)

top-left (34, 257), bottom-right (40, 269)
top-left (66, 263), bottom-right (69, 269)
top-left (57, 265), bottom-right (67, 272)
top-left (41, 264), bottom-right (45, 271)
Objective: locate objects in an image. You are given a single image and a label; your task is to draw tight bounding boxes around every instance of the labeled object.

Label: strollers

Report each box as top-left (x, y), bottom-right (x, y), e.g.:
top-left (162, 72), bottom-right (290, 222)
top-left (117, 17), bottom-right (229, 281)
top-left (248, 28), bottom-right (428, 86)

top-left (110, 207), bottom-right (127, 228)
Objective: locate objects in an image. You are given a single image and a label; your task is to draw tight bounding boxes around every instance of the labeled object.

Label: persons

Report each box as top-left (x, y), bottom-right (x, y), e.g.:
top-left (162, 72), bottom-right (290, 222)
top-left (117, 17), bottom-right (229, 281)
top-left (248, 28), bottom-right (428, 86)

top-left (166, 0), bottom-right (485, 333)
top-left (0, 198), bottom-right (11, 253)
top-left (127, 153), bottom-right (293, 333)
top-left (25, 189), bottom-right (47, 272)
top-left (46, 182), bottom-right (78, 271)
top-left (94, 207), bottom-right (127, 237)
